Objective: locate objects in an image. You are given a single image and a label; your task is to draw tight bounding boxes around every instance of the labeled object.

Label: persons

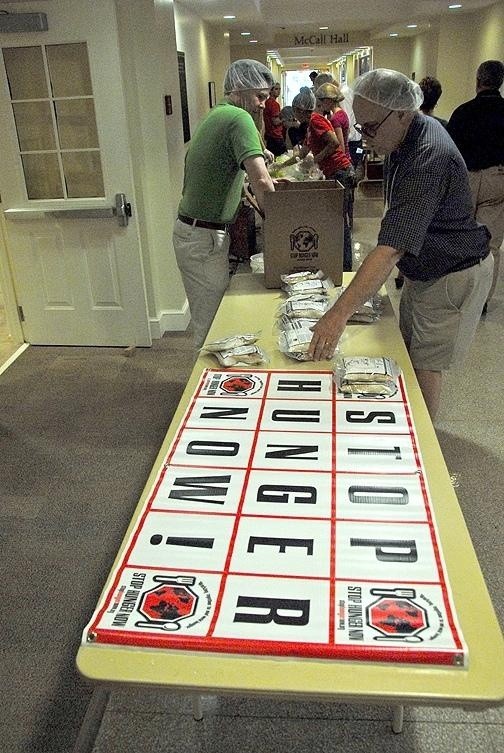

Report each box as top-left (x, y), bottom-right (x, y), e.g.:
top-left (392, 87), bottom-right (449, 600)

top-left (307, 67), bottom-right (495, 423)
top-left (444, 59), bottom-right (504, 315)
top-left (227, 70), bottom-right (364, 271)
top-left (418, 75), bottom-right (447, 127)
top-left (172, 58), bottom-right (276, 364)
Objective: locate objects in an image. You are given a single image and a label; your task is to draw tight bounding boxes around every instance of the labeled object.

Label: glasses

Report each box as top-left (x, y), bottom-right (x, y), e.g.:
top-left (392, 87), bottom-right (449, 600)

top-left (353, 110), bottom-right (393, 138)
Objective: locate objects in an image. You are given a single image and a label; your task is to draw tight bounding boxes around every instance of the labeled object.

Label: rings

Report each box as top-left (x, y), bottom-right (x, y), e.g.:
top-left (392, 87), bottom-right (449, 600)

top-left (325, 342), bottom-right (331, 346)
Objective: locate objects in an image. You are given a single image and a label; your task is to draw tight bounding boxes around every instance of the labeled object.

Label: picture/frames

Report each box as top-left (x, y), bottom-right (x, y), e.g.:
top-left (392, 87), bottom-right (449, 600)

top-left (208, 81), bottom-right (217, 108)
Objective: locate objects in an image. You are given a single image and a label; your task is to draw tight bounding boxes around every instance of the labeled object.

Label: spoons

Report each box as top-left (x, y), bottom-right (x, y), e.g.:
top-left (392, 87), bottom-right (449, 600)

top-left (221, 392), bottom-right (247, 396)
top-left (373, 636), bottom-right (423, 643)
top-left (357, 395), bottom-right (386, 399)
top-left (135, 621), bottom-right (181, 631)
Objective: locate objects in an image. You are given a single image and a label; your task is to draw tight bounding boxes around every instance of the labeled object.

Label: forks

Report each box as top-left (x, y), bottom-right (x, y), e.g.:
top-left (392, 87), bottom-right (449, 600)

top-left (370, 588), bottom-right (416, 598)
top-left (153, 575), bottom-right (196, 585)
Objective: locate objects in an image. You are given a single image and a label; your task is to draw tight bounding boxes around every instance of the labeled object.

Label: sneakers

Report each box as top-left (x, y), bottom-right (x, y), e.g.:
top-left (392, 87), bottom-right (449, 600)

top-left (394, 276), bottom-right (405, 290)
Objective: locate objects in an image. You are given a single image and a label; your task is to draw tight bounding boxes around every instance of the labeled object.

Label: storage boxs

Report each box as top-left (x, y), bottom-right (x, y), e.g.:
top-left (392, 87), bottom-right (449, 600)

top-left (262, 178), bottom-right (346, 288)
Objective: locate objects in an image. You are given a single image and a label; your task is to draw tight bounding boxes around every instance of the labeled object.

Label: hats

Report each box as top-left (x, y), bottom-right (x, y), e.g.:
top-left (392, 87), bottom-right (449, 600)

top-left (315, 73), bottom-right (339, 87)
top-left (316, 83), bottom-right (344, 101)
top-left (223, 58), bottom-right (274, 94)
top-left (292, 87), bottom-right (317, 110)
top-left (351, 68), bottom-right (424, 111)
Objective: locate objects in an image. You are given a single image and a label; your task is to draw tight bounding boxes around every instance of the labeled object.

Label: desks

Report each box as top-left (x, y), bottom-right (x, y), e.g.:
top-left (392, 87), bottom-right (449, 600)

top-left (356, 137), bottom-right (385, 192)
top-left (76, 272), bottom-right (504, 734)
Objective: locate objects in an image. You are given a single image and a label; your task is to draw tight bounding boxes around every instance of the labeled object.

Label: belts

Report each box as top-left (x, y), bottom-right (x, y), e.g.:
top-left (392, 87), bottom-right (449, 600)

top-left (178, 214), bottom-right (230, 231)
top-left (439, 251), bottom-right (490, 275)
